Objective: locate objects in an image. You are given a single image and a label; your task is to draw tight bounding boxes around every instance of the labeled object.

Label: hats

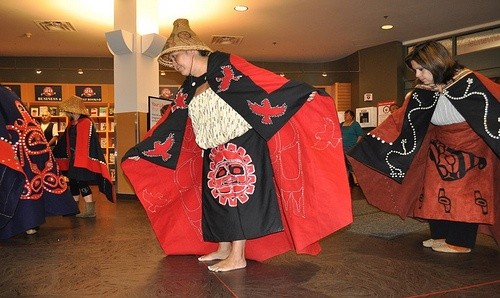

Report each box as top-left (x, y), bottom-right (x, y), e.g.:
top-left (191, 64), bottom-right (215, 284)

top-left (157, 18), bottom-right (213, 67)
top-left (59, 96), bottom-right (90, 116)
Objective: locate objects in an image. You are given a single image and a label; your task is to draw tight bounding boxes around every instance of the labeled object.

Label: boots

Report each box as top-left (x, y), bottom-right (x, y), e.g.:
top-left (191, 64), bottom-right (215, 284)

top-left (75, 201), bottom-right (96, 217)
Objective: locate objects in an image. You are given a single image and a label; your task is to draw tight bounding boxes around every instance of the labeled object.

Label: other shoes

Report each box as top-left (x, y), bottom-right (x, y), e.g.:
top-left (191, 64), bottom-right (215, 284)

top-left (26, 227), bottom-right (37, 234)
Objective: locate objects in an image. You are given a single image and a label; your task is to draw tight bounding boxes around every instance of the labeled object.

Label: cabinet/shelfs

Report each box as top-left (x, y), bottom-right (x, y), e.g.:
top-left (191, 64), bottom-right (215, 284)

top-left (28, 103), bottom-right (115, 184)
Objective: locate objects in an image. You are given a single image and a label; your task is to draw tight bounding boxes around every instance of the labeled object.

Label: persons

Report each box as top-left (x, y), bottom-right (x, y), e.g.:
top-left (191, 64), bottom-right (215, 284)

top-left (0, 84), bottom-right (81, 241)
top-left (159, 104), bottom-right (170, 118)
top-left (39, 111), bottom-right (59, 151)
top-left (121, 18), bottom-right (353, 273)
top-left (339, 111), bottom-right (363, 187)
top-left (51, 95), bottom-right (114, 218)
top-left (344, 40), bottom-right (500, 253)
top-left (388, 103), bottom-right (398, 114)
